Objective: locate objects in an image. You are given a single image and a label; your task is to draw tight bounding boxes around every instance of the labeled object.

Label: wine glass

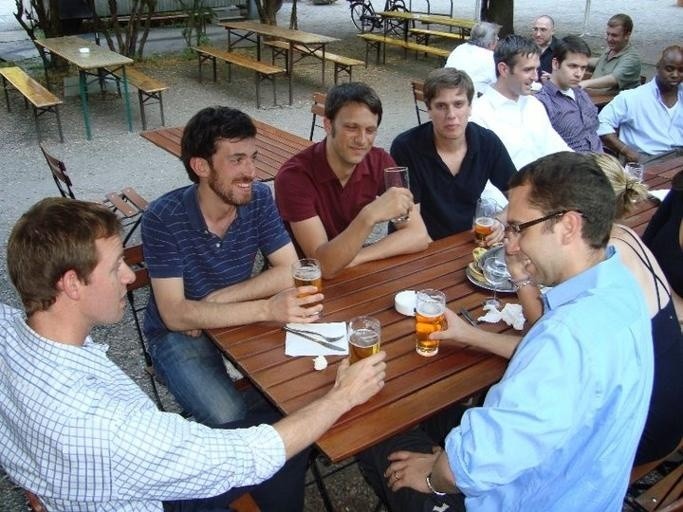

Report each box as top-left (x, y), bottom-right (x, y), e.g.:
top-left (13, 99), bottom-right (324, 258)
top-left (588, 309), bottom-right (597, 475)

top-left (481, 245), bottom-right (506, 309)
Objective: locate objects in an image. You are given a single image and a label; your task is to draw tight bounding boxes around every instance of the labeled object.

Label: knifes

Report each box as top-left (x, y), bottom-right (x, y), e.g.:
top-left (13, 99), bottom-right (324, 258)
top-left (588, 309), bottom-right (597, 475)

top-left (280, 325), bottom-right (346, 352)
top-left (458, 306), bottom-right (483, 333)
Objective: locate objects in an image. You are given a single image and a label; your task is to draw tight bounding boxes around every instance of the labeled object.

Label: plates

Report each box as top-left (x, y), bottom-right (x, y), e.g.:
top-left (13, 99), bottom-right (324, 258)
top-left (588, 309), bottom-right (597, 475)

top-left (462, 264), bottom-right (521, 294)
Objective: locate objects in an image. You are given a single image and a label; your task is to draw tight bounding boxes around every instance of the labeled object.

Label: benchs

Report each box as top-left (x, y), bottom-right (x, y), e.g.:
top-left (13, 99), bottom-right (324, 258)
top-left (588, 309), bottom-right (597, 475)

top-left (96, 11), bottom-right (216, 32)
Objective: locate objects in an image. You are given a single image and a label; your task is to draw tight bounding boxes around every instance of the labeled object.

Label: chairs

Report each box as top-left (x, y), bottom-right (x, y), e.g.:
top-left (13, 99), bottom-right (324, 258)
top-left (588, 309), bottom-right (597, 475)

top-left (357, 31), bottom-right (452, 70)
top-left (36, 141), bottom-right (150, 272)
top-left (188, 43), bottom-right (286, 110)
top-left (115, 238), bottom-right (338, 512)
top-left (409, 27), bottom-right (470, 57)
top-left (406, 80), bottom-right (431, 125)
top-left (266, 40), bottom-right (365, 88)
top-left (98, 59), bottom-right (169, 126)
top-left (0, 65), bottom-right (66, 145)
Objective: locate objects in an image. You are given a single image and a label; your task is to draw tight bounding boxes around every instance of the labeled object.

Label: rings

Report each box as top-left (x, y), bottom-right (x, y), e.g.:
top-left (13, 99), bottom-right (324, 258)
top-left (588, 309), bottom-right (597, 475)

top-left (393, 472), bottom-right (399, 480)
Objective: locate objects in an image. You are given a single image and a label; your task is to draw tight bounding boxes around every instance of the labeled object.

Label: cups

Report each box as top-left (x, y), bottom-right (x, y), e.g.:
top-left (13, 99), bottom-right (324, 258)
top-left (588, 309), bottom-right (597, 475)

top-left (381, 166), bottom-right (411, 226)
top-left (345, 316), bottom-right (381, 366)
top-left (414, 288), bottom-right (444, 357)
top-left (289, 257), bottom-right (325, 314)
top-left (473, 198), bottom-right (497, 250)
top-left (622, 162), bottom-right (645, 205)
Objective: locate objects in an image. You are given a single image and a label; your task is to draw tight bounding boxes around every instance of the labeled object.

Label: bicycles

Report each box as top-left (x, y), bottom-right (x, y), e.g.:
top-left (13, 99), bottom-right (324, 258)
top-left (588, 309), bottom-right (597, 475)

top-left (348, 0), bottom-right (414, 39)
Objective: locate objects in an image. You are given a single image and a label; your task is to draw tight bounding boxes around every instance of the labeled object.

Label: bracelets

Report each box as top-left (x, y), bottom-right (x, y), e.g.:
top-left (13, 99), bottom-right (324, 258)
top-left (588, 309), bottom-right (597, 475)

top-left (425, 473), bottom-right (447, 496)
top-left (620, 145), bottom-right (627, 152)
top-left (512, 279), bottom-right (531, 288)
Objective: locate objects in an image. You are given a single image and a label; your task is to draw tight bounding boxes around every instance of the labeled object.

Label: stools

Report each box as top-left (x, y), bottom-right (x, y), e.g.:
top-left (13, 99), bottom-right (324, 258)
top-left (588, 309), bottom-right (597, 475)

top-left (21, 483), bottom-right (260, 512)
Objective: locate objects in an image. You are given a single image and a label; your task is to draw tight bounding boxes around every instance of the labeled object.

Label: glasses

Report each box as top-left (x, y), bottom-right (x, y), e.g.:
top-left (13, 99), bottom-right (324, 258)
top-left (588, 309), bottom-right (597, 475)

top-left (504, 210), bottom-right (587, 239)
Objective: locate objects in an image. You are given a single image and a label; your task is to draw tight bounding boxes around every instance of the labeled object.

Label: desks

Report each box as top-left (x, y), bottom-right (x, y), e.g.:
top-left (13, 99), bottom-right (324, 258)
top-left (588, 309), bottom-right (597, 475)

top-left (196, 151), bottom-right (681, 510)
top-left (580, 89), bottom-right (613, 114)
top-left (138, 119), bottom-right (316, 186)
top-left (374, 9), bottom-right (478, 61)
top-left (31, 34), bottom-right (133, 138)
top-left (215, 21), bottom-right (339, 105)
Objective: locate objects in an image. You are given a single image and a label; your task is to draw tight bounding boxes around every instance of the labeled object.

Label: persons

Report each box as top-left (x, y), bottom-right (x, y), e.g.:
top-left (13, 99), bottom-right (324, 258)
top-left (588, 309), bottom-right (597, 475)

top-left (139, 105), bottom-right (301, 426)
top-left (531, 14), bottom-right (563, 85)
top-left (639, 170), bottom-right (682, 301)
top-left (595, 45), bottom-right (682, 168)
top-left (379, 150), bottom-right (656, 511)
top-left (466, 35), bottom-right (574, 173)
top-left (273, 81), bottom-right (429, 282)
top-left (442, 21), bottom-right (500, 98)
top-left (577, 13), bottom-right (643, 92)
top-left (534, 35), bottom-right (605, 154)
top-left (503, 151), bottom-right (682, 469)
top-left (389, 67), bottom-right (520, 244)
top-left (0, 198), bottom-right (387, 511)
